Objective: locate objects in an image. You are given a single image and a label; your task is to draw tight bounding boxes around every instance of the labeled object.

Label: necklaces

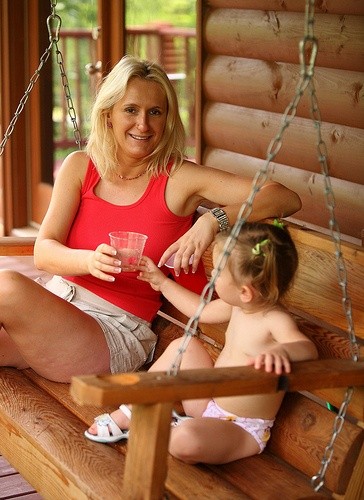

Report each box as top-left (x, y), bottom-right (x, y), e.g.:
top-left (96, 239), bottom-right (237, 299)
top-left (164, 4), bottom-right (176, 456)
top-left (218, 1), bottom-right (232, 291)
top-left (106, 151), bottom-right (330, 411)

top-left (114, 162), bottom-right (147, 181)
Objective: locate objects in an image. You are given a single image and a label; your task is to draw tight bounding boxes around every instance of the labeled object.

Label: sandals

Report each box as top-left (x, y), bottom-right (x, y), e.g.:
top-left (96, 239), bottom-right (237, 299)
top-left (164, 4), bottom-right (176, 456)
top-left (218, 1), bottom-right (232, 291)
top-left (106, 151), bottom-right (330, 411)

top-left (170, 409), bottom-right (193, 428)
top-left (84, 403), bottom-right (131, 443)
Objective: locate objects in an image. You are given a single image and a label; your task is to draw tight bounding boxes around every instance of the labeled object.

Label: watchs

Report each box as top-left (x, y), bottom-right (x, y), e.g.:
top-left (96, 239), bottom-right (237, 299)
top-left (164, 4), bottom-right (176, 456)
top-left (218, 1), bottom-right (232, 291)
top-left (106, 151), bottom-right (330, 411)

top-left (210, 208), bottom-right (231, 233)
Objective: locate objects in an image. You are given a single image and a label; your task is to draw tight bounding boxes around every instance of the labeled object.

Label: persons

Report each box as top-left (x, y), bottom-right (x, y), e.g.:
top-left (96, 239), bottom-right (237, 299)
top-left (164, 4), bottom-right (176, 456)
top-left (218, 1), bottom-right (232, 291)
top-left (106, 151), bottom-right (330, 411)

top-left (0, 55), bottom-right (303, 384)
top-left (84, 217), bottom-right (319, 463)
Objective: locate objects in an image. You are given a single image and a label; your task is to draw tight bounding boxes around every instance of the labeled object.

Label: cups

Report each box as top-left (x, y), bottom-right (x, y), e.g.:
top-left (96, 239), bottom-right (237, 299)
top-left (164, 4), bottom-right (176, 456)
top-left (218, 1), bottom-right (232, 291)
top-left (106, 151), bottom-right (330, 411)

top-left (164, 252), bottom-right (209, 299)
top-left (107, 231), bottom-right (148, 272)
